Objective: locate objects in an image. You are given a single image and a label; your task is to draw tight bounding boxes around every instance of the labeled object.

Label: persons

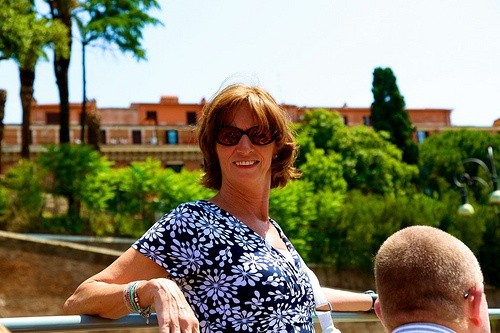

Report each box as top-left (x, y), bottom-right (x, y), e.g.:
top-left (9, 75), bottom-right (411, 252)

top-left (372, 225), bottom-right (491, 333)
top-left (63, 82), bottom-right (379, 333)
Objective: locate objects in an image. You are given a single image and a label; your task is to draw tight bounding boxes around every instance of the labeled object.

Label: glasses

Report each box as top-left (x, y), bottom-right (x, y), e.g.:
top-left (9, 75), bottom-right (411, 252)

top-left (213, 124), bottom-right (278, 147)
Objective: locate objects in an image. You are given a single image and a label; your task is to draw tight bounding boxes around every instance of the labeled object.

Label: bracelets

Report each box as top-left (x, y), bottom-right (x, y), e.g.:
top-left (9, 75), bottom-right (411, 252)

top-left (364, 289), bottom-right (378, 312)
top-left (123, 279), bottom-right (151, 325)
